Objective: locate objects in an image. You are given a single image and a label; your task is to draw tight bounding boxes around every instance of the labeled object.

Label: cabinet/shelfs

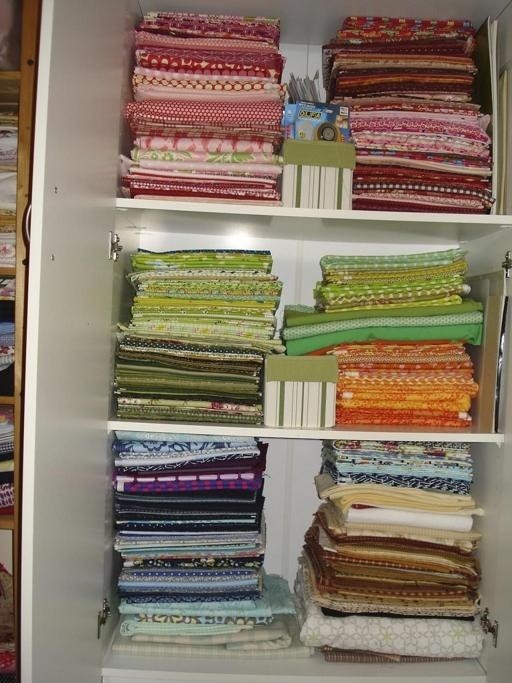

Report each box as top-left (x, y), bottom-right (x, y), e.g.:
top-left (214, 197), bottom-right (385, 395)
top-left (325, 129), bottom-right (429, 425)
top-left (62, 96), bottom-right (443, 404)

top-left (0, 1), bottom-right (40, 681)
top-left (20, 0), bottom-right (511, 682)
